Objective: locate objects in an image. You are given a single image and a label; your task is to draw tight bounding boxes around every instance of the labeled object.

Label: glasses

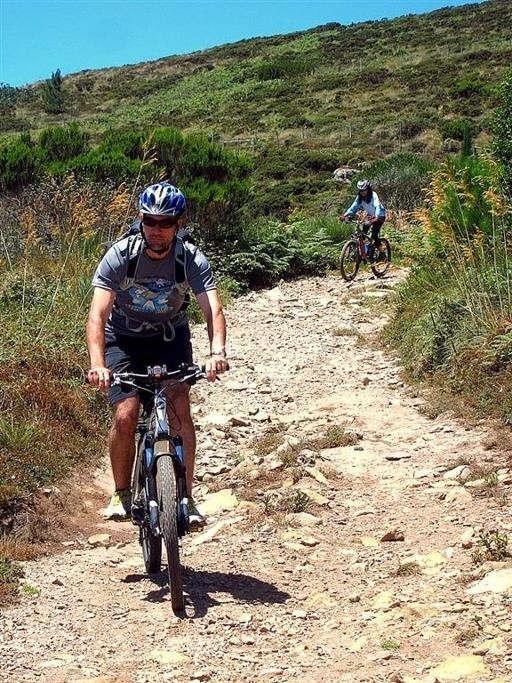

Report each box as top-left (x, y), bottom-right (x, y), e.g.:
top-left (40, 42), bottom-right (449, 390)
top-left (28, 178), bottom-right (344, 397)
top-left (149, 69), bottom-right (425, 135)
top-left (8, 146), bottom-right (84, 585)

top-left (142, 217), bottom-right (177, 228)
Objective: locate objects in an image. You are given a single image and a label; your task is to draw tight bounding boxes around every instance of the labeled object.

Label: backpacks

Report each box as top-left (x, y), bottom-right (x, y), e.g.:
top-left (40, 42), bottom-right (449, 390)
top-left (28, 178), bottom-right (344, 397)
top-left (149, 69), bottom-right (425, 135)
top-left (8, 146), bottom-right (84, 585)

top-left (111, 222), bottom-right (195, 293)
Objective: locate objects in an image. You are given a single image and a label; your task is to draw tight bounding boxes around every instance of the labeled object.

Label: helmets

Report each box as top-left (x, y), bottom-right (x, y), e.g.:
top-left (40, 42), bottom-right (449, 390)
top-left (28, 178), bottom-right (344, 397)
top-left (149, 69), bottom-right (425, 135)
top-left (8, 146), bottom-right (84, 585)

top-left (356, 178), bottom-right (371, 191)
top-left (138, 182), bottom-right (186, 217)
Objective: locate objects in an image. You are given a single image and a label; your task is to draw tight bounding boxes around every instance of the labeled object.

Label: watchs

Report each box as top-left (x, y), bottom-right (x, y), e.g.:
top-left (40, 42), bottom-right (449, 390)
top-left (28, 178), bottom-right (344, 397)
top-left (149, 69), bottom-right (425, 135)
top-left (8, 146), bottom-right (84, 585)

top-left (210, 348), bottom-right (227, 357)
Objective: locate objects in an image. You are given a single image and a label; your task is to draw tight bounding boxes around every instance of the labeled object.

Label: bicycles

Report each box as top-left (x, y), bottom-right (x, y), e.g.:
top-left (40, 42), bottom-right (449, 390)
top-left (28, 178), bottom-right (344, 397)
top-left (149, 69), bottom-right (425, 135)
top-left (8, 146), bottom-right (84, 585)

top-left (339, 212), bottom-right (391, 280)
top-left (85, 357), bottom-right (230, 610)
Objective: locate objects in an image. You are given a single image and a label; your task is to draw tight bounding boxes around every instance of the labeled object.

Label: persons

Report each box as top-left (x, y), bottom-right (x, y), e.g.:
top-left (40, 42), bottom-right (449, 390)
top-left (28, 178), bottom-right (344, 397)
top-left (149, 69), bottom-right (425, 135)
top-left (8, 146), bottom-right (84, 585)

top-left (87, 183), bottom-right (230, 525)
top-left (339, 180), bottom-right (386, 260)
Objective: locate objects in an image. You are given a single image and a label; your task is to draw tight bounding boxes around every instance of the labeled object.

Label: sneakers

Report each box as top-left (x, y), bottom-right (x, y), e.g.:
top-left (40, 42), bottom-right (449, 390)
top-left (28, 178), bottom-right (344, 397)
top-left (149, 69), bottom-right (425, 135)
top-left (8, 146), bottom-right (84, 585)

top-left (178, 496), bottom-right (207, 527)
top-left (103, 487), bottom-right (131, 520)
top-left (373, 252), bottom-right (387, 264)
